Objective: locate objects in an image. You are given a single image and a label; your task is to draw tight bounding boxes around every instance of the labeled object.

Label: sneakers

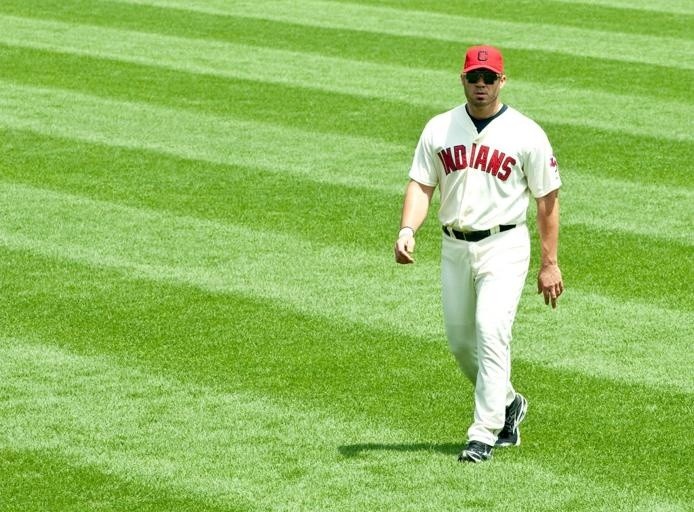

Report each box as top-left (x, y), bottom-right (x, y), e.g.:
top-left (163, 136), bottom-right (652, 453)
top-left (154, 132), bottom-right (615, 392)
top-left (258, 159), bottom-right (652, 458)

top-left (458, 440), bottom-right (493, 461)
top-left (496, 395), bottom-right (527, 447)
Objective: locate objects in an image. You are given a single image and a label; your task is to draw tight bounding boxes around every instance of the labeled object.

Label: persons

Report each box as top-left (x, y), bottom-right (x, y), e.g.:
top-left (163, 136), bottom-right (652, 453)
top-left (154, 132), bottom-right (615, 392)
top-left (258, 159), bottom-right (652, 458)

top-left (394, 44), bottom-right (565, 465)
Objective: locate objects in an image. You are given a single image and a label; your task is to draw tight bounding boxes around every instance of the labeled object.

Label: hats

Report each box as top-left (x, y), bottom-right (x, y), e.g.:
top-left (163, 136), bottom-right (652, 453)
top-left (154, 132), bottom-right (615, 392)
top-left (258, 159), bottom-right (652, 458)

top-left (465, 45), bottom-right (504, 75)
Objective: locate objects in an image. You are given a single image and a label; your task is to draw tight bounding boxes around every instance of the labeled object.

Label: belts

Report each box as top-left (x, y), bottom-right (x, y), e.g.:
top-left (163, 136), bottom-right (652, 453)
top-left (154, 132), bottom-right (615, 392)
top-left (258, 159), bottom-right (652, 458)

top-left (442, 223), bottom-right (516, 242)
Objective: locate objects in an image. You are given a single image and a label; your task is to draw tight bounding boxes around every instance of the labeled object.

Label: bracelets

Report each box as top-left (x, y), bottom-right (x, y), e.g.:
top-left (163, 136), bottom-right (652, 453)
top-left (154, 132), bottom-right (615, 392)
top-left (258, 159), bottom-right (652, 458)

top-left (398, 225), bottom-right (415, 239)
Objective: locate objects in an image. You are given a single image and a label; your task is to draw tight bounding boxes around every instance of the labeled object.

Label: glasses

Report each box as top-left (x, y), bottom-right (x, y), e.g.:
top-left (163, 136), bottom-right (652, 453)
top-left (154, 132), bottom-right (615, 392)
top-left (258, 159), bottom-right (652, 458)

top-left (465, 71), bottom-right (501, 85)
